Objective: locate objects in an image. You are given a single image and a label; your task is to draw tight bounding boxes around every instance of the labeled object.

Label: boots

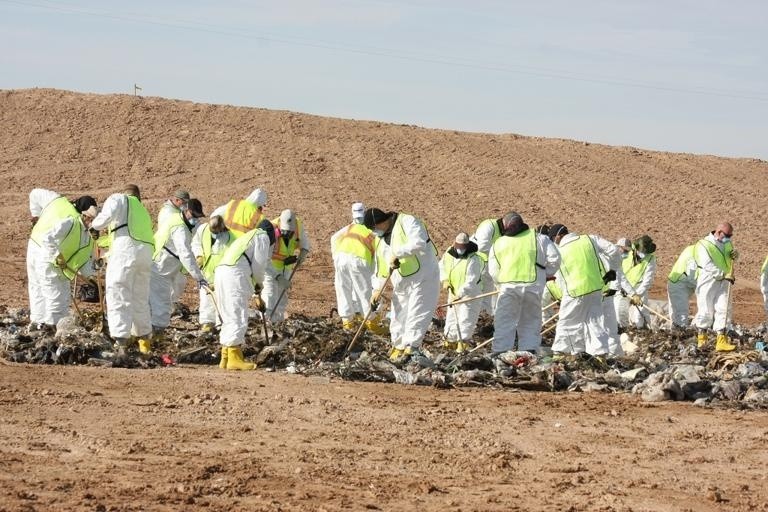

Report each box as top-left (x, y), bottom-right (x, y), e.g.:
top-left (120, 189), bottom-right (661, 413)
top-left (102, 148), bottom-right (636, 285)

top-left (390, 346), bottom-right (419, 362)
top-left (138, 338), bottom-right (151, 354)
top-left (219, 344), bottom-right (258, 370)
top-left (343, 313), bottom-right (390, 336)
top-left (445, 340), bottom-right (473, 352)
top-left (696, 334), bottom-right (708, 347)
top-left (716, 334), bottom-right (736, 351)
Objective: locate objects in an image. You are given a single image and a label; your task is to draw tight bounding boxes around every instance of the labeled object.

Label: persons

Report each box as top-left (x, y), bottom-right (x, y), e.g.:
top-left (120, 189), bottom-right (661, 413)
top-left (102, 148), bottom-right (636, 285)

top-left (694, 223), bottom-right (739, 353)
top-left (667, 230), bottom-right (715, 340)
top-left (438, 211), bottom-right (656, 363)
top-left (89, 184), bottom-right (155, 357)
top-left (149, 187), bottom-right (310, 371)
top-left (27, 188), bottom-right (98, 329)
top-left (330, 202), bottom-right (441, 363)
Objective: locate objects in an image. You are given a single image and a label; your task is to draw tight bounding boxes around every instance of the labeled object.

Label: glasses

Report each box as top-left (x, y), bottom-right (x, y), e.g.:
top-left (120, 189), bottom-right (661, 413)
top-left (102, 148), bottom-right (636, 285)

top-left (722, 232), bottom-right (733, 238)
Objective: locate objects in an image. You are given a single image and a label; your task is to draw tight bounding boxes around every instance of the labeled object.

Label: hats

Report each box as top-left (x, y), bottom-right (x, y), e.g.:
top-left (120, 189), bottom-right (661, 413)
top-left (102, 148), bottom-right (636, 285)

top-left (454, 232), bottom-right (469, 249)
top-left (280, 209), bottom-right (296, 231)
top-left (633, 236), bottom-right (656, 253)
top-left (175, 189), bottom-right (205, 216)
top-left (538, 224), bottom-right (567, 238)
top-left (73, 196), bottom-right (101, 218)
top-left (352, 203), bottom-right (387, 228)
top-left (123, 183), bottom-right (141, 200)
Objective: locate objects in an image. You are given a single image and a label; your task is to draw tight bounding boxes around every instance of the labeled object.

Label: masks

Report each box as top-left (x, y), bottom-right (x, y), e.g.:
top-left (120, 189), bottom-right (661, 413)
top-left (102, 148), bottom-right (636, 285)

top-left (211, 233), bottom-right (221, 239)
top-left (638, 252), bottom-right (646, 259)
top-left (722, 238), bottom-right (729, 243)
top-left (189, 218), bottom-right (199, 225)
top-left (457, 249), bottom-right (466, 255)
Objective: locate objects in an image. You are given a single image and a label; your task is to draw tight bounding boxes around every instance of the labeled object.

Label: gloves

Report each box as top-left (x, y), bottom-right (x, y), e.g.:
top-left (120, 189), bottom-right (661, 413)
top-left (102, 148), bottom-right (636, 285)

top-left (389, 258), bottom-right (400, 274)
top-left (602, 269), bottom-right (616, 284)
top-left (197, 280), bottom-right (210, 290)
top-left (56, 253), bottom-right (66, 269)
top-left (450, 295), bottom-right (460, 308)
top-left (282, 256), bottom-right (300, 265)
top-left (443, 280), bottom-right (454, 290)
top-left (277, 274), bottom-right (290, 288)
top-left (254, 282), bottom-right (265, 296)
top-left (731, 249), bottom-right (739, 259)
top-left (370, 291), bottom-right (383, 311)
top-left (88, 226), bottom-right (101, 240)
top-left (603, 289), bottom-right (617, 297)
top-left (630, 296), bottom-right (643, 306)
top-left (724, 273), bottom-right (735, 285)
